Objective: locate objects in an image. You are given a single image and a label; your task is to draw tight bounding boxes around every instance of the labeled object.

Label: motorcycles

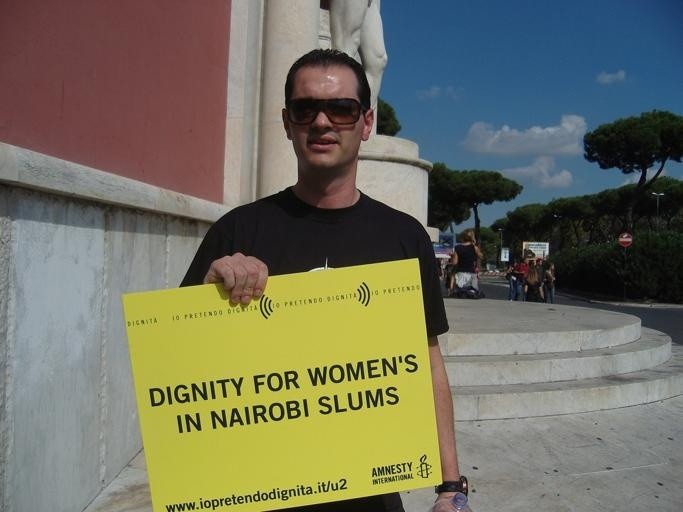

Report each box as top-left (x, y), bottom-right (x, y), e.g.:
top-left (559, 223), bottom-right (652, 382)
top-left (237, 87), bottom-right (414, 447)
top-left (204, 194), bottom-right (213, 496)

top-left (442, 254), bottom-right (483, 298)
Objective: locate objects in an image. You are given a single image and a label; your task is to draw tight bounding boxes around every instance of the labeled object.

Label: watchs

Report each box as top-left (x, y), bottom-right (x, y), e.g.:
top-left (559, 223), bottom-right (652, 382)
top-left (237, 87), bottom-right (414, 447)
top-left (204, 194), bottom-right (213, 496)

top-left (434, 475), bottom-right (469, 498)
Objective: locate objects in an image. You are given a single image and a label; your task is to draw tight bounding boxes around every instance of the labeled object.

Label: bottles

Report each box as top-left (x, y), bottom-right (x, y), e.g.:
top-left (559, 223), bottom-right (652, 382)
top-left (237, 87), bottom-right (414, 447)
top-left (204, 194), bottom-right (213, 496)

top-left (447, 492), bottom-right (469, 512)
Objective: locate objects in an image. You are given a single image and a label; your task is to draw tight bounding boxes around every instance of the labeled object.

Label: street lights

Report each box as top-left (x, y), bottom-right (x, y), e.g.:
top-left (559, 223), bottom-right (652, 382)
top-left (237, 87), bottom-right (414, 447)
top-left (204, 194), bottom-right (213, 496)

top-left (553, 214), bottom-right (562, 219)
top-left (498, 227), bottom-right (505, 273)
top-left (650, 189), bottom-right (664, 236)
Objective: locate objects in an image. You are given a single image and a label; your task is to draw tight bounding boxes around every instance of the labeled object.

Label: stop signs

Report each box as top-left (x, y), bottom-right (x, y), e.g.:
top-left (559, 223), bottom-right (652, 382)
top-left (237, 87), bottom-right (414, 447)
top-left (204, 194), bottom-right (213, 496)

top-left (618, 233), bottom-right (633, 248)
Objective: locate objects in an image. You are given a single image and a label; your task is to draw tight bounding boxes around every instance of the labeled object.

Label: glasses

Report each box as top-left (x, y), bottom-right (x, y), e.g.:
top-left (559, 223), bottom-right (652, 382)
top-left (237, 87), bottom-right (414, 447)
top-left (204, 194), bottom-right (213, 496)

top-left (285, 98), bottom-right (360, 125)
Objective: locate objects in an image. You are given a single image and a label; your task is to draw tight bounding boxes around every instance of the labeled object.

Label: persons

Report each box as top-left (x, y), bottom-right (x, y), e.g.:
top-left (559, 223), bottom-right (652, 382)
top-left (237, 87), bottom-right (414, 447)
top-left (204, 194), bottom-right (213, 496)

top-left (505, 257), bottom-right (558, 304)
top-left (329, 0), bottom-right (389, 133)
top-left (179, 48), bottom-right (471, 511)
top-left (444, 229), bottom-right (484, 299)
